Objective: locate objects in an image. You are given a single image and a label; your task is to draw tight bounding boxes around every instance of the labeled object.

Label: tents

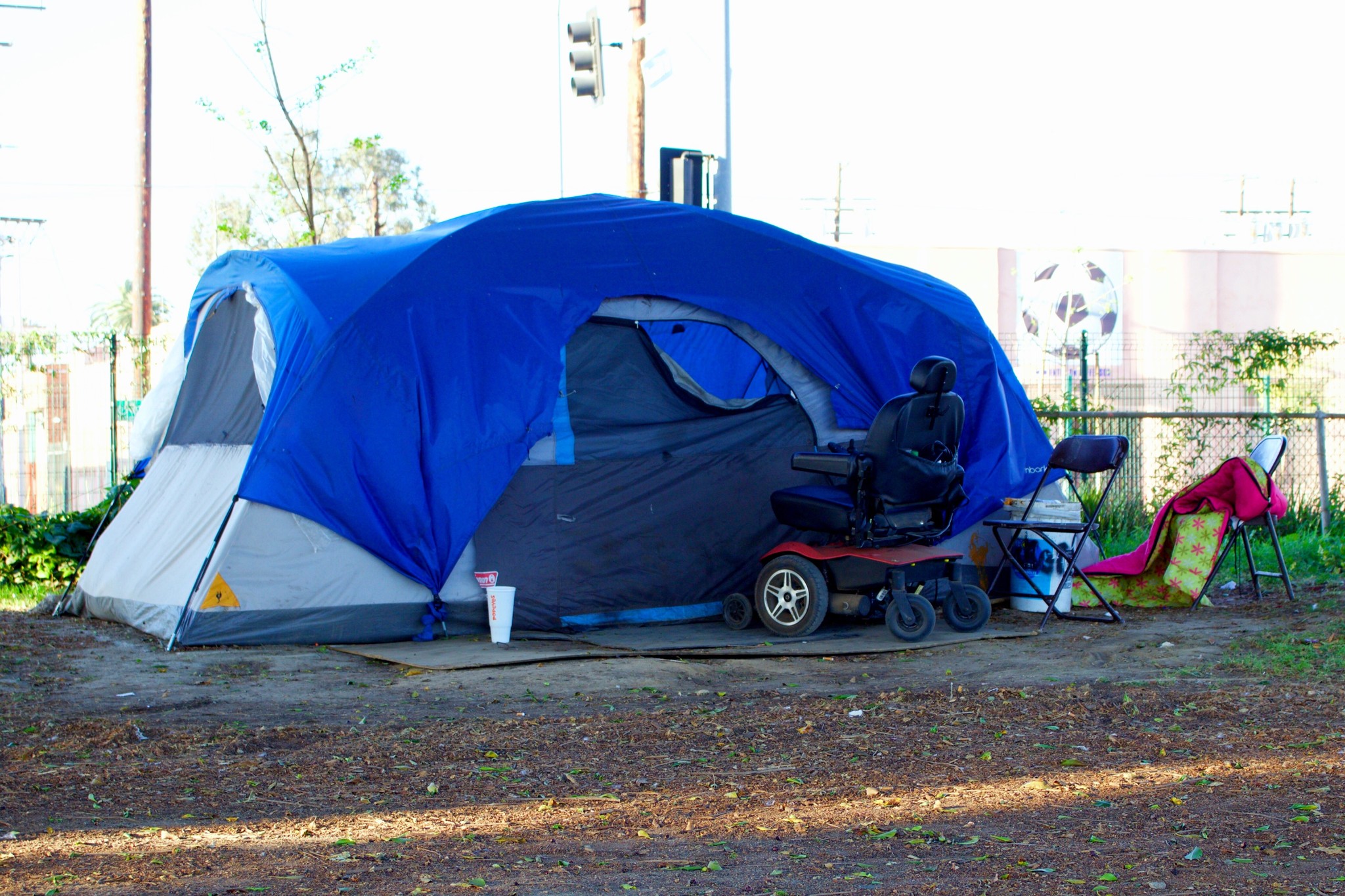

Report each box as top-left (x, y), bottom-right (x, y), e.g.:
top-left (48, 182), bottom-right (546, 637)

top-left (51, 192), bottom-right (1111, 653)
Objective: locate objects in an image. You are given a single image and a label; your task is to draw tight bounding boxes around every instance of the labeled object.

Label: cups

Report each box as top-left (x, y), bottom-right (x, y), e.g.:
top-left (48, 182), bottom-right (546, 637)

top-left (486, 586), bottom-right (516, 643)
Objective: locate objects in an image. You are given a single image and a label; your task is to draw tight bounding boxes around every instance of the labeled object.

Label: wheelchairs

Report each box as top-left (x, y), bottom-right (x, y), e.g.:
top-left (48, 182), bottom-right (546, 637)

top-left (722, 355), bottom-right (994, 639)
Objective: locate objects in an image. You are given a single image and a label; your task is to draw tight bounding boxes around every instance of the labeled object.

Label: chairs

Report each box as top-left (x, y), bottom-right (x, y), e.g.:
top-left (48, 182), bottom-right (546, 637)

top-left (1161, 434), bottom-right (1298, 615)
top-left (981, 432), bottom-right (1132, 634)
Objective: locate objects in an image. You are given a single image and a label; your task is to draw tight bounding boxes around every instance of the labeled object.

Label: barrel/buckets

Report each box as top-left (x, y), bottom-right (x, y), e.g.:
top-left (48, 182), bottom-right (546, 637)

top-left (1004, 498), bottom-right (1082, 613)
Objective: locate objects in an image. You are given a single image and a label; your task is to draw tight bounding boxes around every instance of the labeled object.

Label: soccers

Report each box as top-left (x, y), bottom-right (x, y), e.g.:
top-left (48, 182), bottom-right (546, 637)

top-left (1019, 258), bottom-right (1118, 360)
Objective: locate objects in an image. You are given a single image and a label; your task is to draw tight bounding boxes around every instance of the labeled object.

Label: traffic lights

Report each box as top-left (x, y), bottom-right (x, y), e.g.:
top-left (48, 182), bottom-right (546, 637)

top-left (567, 14), bottom-right (607, 101)
